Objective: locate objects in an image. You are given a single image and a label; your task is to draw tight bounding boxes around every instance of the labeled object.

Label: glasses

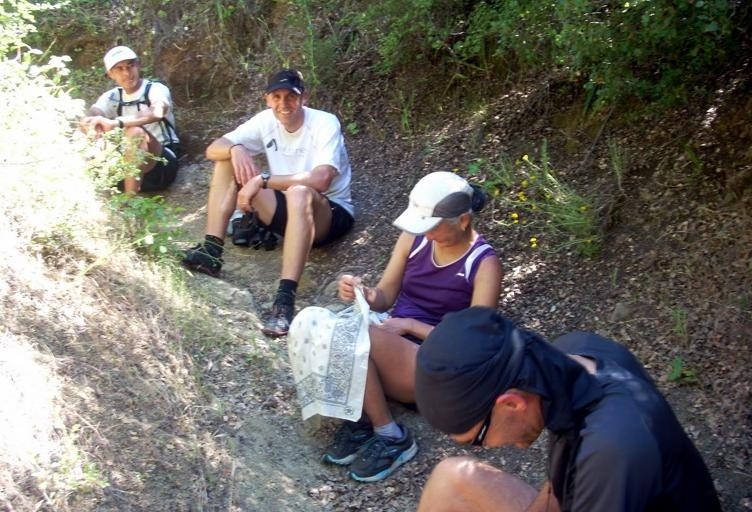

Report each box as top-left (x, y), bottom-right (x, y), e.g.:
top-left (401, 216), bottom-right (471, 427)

top-left (467, 417), bottom-right (491, 453)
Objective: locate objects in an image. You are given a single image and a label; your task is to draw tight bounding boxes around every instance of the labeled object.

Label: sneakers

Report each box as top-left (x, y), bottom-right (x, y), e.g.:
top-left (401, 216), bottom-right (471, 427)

top-left (322, 412), bottom-right (376, 466)
top-left (263, 301), bottom-right (297, 335)
top-left (351, 425), bottom-right (419, 482)
top-left (178, 243), bottom-right (222, 277)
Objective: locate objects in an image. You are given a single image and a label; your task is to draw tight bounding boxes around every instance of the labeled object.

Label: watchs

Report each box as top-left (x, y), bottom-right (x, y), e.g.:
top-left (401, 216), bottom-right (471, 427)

top-left (261, 170), bottom-right (271, 189)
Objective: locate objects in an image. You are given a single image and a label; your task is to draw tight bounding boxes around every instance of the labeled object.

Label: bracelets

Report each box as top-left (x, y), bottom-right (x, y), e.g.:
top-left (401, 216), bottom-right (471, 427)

top-left (229, 143), bottom-right (243, 157)
top-left (116, 118), bottom-right (124, 128)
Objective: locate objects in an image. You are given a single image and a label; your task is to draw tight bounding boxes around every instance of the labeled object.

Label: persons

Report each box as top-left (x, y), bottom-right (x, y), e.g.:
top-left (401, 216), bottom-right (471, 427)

top-left (171, 66), bottom-right (357, 337)
top-left (320, 170), bottom-right (503, 484)
top-left (414, 305), bottom-right (722, 512)
top-left (79, 44), bottom-right (181, 207)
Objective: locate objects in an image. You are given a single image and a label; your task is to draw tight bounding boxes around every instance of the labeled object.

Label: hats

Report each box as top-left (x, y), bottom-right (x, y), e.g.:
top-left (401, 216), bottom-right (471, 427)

top-left (267, 69), bottom-right (304, 95)
top-left (103, 46), bottom-right (137, 72)
top-left (391, 171), bottom-right (474, 236)
